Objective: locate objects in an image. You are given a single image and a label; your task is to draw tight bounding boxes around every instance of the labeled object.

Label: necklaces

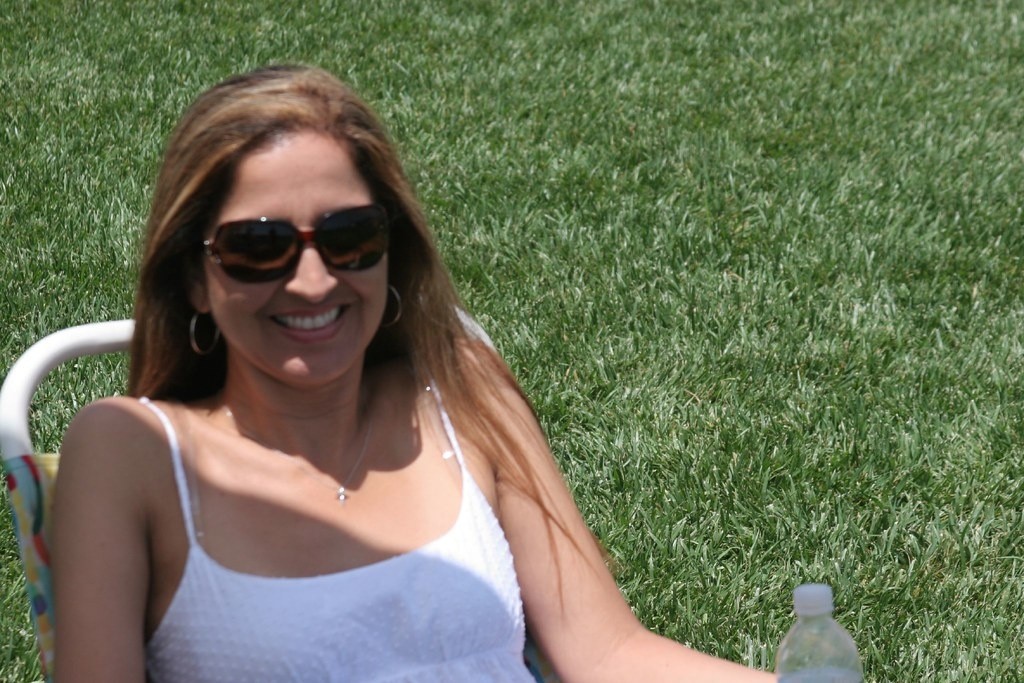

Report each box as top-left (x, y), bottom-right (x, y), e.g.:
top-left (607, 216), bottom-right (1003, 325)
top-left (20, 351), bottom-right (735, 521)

top-left (221, 393), bottom-right (372, 502)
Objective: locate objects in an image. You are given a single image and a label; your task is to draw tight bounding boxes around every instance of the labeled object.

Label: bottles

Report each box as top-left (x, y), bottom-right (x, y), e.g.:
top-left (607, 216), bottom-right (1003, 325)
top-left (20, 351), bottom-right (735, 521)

top-left (773, 582), bottom-right (863, 683)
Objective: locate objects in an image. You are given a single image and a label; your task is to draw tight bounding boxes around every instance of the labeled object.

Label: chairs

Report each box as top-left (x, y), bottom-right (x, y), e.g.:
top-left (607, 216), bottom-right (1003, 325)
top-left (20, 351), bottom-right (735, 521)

top-left (1, 295), bottom-right (498, 683)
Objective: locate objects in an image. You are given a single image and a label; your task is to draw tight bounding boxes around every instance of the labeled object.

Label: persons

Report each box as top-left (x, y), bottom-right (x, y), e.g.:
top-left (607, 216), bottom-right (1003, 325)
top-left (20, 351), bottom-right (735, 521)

top-left (50, 63), bottom-right (780, 683)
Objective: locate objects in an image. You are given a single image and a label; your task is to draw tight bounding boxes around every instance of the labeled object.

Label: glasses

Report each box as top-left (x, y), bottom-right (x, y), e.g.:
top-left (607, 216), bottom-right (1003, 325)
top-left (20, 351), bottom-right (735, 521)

top-left (201, 202), bottom-right (390, 284)
